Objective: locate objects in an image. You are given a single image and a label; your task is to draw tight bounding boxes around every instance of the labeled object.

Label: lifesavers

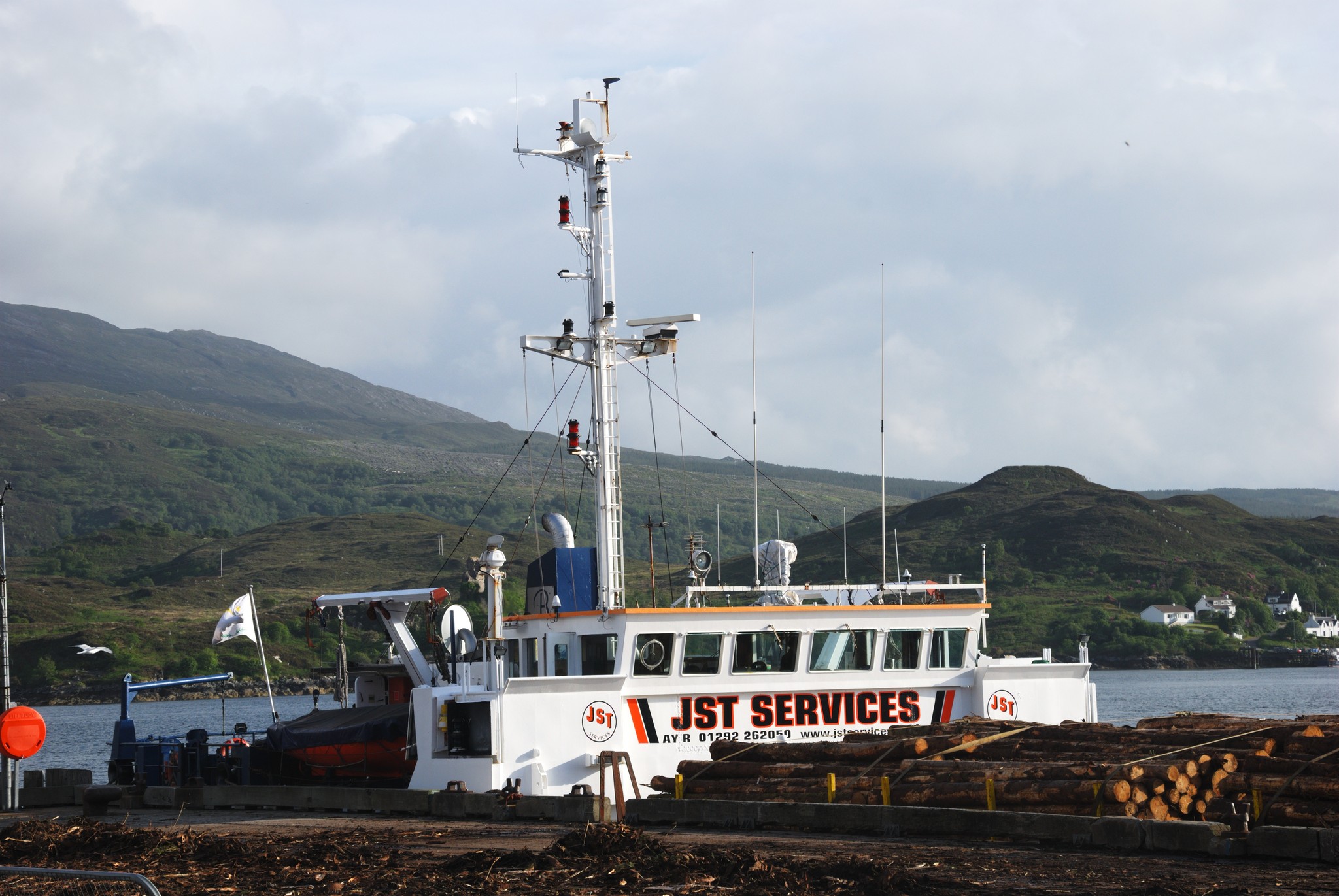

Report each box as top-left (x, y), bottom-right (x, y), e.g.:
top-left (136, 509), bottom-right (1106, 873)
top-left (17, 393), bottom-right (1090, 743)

top-left (219, 738), bottom-right (251, 759)
top-left (164, 751), bottom-right (178, 786)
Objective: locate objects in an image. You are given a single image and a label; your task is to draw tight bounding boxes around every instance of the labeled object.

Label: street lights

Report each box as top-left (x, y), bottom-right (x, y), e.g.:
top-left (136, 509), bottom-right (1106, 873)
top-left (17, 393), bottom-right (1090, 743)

top-left (1292, 619), bottom-right (1296, 648)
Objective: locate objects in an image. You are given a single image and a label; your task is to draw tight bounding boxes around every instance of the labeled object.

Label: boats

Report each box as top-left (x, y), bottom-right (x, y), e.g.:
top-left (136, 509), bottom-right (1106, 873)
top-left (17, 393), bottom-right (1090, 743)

top-left (107, 76), bottom-right (1102, 794)
